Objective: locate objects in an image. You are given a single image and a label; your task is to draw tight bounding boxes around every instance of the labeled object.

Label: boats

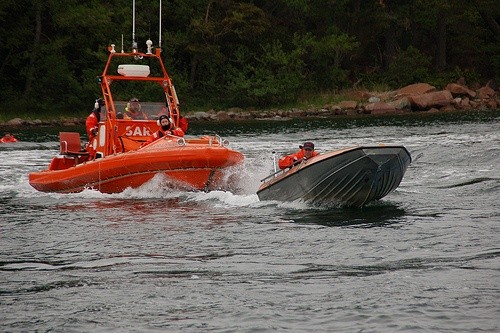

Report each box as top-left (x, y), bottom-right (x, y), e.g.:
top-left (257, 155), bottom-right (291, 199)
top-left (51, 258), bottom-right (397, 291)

top-left (256, 145), bottom-right (413, 207)
top-left (29, 0), bottom-right (244, 192)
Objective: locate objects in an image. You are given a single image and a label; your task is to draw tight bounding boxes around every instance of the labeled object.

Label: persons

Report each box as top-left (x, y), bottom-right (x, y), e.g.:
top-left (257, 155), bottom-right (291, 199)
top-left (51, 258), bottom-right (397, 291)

top-left (85, 99), bottom-right (105, 140)
top-left (279, 142), bottom-right (319, 170)
top-left (0, 132), bottom-right (19, 144)
top-left (137, 115), bottom-right (184, 149)
top-left (123, 98), bottom-right (148, 120)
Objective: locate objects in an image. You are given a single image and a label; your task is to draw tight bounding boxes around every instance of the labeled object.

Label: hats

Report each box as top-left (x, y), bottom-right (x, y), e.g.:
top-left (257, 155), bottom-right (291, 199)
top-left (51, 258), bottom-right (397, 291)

top-left (304, 142), bottom-right (314, 151)
top-left (160, 115), bottom-right (169, 120)
